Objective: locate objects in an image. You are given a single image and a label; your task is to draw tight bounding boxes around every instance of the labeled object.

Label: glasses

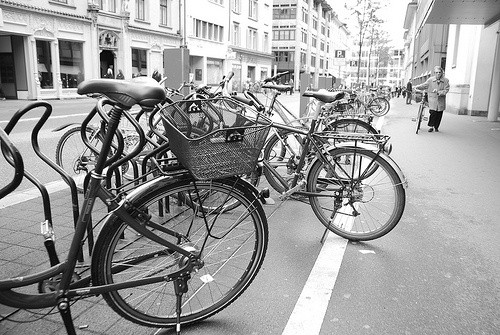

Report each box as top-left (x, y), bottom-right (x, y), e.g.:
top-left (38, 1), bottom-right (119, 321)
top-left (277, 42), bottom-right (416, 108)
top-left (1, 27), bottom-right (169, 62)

top-left (436, 72), bottom-right (441, 73)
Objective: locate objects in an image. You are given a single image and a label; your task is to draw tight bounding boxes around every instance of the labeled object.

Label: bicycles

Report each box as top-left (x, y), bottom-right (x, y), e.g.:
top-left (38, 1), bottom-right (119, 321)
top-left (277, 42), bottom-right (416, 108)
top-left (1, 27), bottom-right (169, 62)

top-left (51, 68), bottom-right (408, 244)
top-left (0, 74), bottom-right (274, 335)
top-left (411, 87), bottom-right (437, 134)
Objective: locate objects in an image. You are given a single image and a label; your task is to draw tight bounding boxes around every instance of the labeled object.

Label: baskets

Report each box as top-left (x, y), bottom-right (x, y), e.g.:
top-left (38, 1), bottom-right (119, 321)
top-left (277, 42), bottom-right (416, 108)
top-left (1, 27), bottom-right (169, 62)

top-left (158, 96), bottom-right (274, 182)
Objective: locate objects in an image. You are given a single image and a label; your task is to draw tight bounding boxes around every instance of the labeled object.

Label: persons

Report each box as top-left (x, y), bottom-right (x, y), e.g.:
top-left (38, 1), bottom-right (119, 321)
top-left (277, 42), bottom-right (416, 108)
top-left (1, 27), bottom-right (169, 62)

top-left (151, 69), bottom-right (162, 83)
top-left (415, 66), bottom-right (451, 133)
top-left (289, 78), bottom-right (294, 95)
top-left (116, 70), bottom-right (124, 79)
top-left (396, 86), bottom-right (407, 98)
top-left (284, 80), bottom-right (289, 95)
top-left (107, 64), bottom-right (114, 79)
top-left (406, 79), bottom-right (412, 104)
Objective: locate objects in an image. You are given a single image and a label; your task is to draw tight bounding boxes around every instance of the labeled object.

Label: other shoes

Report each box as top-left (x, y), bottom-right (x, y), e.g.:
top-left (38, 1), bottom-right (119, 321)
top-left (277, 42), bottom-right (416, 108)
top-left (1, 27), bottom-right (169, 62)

top-left (406, 103), bottom-right (412, 105)
top-left (428, 128), bottom-right (433, 132)
top-left (434, 127), bottom-right (438, 132)
